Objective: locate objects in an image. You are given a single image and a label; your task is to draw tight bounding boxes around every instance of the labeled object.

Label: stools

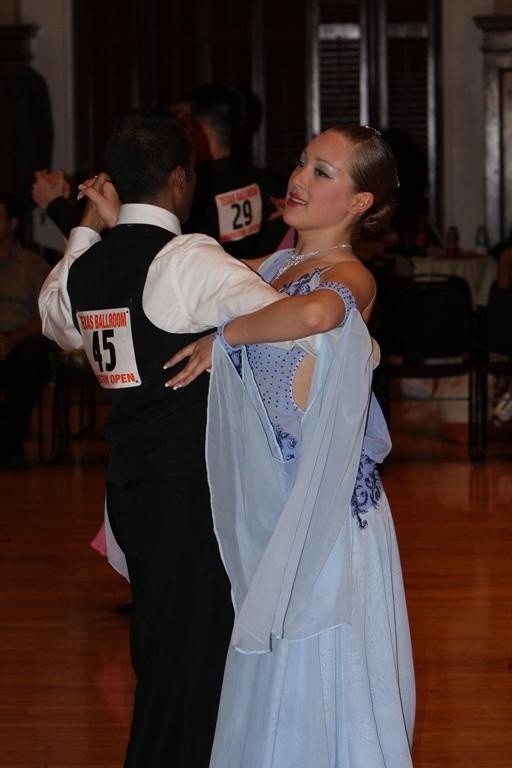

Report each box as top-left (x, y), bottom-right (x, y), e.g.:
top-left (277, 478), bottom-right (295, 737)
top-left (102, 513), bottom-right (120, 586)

top-left (24, 352), bottom-right (95, 469)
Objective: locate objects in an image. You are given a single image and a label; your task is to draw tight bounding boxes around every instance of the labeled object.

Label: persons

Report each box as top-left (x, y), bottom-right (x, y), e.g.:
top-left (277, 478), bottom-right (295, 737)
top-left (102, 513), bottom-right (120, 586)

top-left (2, 89), bottom-right (512, 619)
top-left (71, 121), bottom-right (422, 768)
top-left (29, 108), bottom-right (385, 768)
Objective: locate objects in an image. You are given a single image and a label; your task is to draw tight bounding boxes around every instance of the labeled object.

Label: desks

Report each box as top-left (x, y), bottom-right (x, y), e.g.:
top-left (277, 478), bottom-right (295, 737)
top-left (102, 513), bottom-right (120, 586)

top-left (360, 254), bottom-right (496, 315)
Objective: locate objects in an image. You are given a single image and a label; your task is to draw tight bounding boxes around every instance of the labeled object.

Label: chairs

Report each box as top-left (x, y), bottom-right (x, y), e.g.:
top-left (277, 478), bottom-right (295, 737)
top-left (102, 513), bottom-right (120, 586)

top-left (366, 273), bottom-right (492, 466)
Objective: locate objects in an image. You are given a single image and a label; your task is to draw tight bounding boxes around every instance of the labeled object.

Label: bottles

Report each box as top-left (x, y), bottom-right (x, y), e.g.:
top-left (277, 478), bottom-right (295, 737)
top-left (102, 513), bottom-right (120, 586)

top-left (473, 224), bottom-right (488, 257)
top-left (443, 225), bottom-right (458, 257)
